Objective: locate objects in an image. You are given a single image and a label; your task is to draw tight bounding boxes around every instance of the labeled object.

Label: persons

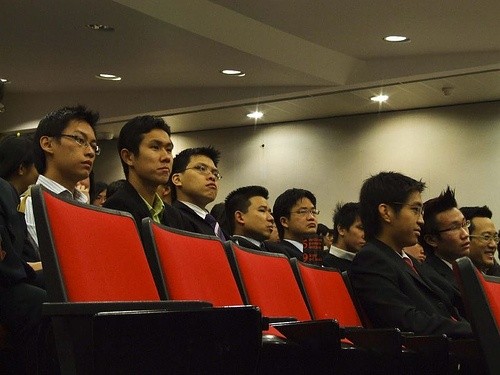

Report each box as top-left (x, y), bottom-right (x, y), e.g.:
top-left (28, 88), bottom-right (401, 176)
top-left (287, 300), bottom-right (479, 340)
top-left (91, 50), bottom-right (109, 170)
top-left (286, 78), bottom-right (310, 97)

top-left (0, 103), bottom-right (500, 375)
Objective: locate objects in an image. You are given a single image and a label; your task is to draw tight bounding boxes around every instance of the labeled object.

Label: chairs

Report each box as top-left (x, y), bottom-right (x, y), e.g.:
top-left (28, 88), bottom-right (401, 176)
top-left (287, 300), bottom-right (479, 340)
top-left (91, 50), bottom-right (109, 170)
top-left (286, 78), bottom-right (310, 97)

top-left (32, 184), bottom-right (500, 375)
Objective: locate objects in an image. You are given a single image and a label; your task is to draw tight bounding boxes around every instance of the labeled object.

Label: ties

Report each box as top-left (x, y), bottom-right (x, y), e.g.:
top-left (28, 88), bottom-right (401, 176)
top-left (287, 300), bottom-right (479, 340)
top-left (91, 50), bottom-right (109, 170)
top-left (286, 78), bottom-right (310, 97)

top-left (205, 214), bottom-right (226, 242)
top-left (261, 243), bottom-right (266, 251)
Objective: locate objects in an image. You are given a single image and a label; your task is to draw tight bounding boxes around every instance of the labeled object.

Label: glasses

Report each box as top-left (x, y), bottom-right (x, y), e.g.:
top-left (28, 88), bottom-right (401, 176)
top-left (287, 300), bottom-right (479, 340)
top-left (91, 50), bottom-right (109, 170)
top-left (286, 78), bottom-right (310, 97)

top-left (470, 235), bottom-right (500, 243)
top-left (61, 134), bottom-right (100, 155)
top-left (290, 209), bottom-right (320, 215)
top-left (439, 220), bottom-right (471, 232)
top-left (386, 202), bottom-right (424, 216)
top-left (178, 166), bottom-right (222, 180)
